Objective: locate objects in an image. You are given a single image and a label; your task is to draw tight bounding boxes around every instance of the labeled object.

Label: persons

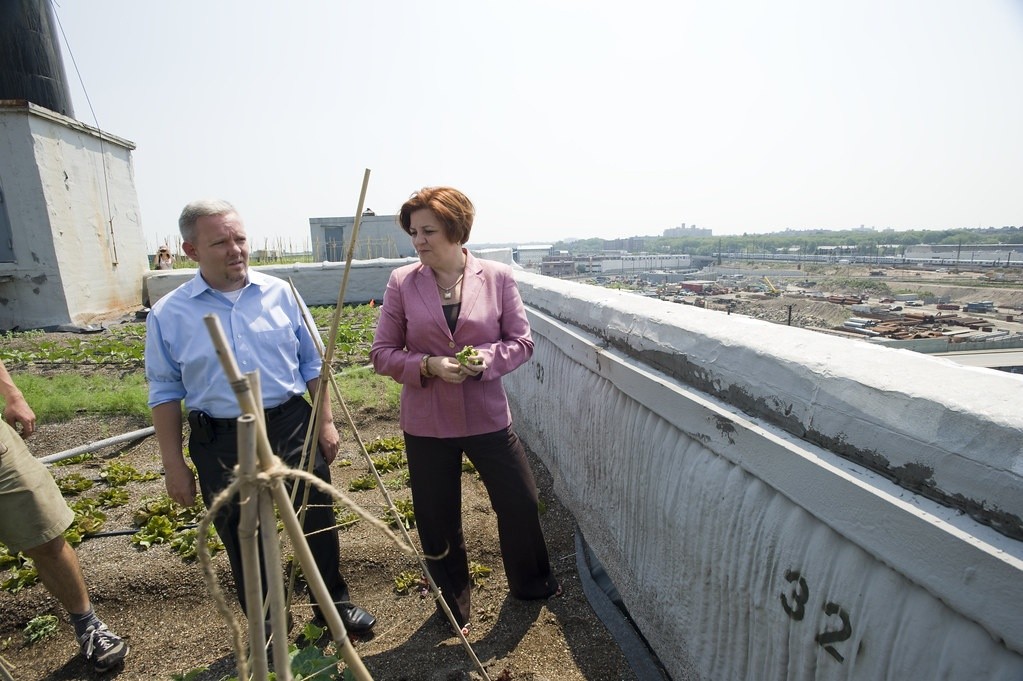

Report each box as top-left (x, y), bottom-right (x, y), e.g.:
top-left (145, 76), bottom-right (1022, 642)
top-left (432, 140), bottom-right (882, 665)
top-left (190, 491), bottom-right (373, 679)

top-left (367, 186), bottom-right (565, 638)
top-left (155, 244), bottom-right (175, 270)
top-left (143, 195), bottom-right (377, 655)
top-left (0, 358), bottom-right (130, 673)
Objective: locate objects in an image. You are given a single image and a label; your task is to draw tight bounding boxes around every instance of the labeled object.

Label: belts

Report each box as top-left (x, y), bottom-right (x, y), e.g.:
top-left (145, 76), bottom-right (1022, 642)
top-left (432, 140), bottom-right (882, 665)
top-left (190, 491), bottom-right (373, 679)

top-left (213, 396), bottom-right (301, 427)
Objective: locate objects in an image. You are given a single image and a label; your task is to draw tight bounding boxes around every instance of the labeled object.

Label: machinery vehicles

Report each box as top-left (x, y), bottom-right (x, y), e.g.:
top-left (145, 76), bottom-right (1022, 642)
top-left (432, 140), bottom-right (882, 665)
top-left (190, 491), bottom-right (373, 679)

top-left (762, 274), bottom-right (776, 295)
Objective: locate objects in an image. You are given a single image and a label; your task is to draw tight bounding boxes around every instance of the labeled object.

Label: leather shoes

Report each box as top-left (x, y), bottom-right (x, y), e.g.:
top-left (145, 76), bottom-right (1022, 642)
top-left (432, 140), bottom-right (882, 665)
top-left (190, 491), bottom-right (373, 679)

top-left (240, 603), bottom-right (294, 637)
top-left (312, 602), bottom-right (376, 631)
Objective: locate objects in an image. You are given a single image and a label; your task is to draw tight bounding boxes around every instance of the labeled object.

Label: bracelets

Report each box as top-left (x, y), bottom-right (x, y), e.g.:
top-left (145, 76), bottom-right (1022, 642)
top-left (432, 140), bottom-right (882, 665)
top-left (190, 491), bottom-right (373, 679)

top-left (421, 355), bottom-right (435, 379)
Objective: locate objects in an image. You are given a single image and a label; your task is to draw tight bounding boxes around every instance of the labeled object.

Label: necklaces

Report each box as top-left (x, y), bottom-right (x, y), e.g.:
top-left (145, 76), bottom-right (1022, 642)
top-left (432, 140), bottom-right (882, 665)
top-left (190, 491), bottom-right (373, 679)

top-left (437, 274), bottom-right (464, 300)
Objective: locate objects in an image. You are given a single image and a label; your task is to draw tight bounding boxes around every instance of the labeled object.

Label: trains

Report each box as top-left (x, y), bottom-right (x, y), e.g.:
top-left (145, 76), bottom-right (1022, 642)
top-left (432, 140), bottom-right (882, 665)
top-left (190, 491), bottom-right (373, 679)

top-left (711, 252), bottom-right (1023, 268)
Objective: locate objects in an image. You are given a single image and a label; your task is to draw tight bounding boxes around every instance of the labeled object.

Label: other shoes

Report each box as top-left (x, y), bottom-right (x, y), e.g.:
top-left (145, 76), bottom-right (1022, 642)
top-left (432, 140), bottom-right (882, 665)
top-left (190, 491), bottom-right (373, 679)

top-left (510, 583), bottom-right (565, 599)
top-left (435, 608), bottom-right (470, 638)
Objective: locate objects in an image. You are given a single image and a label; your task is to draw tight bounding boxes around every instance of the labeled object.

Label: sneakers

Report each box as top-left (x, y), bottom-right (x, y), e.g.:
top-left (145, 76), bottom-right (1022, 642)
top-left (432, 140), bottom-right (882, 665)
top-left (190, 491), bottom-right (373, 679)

top-left (74, 620), bottom-right (130, 671)
